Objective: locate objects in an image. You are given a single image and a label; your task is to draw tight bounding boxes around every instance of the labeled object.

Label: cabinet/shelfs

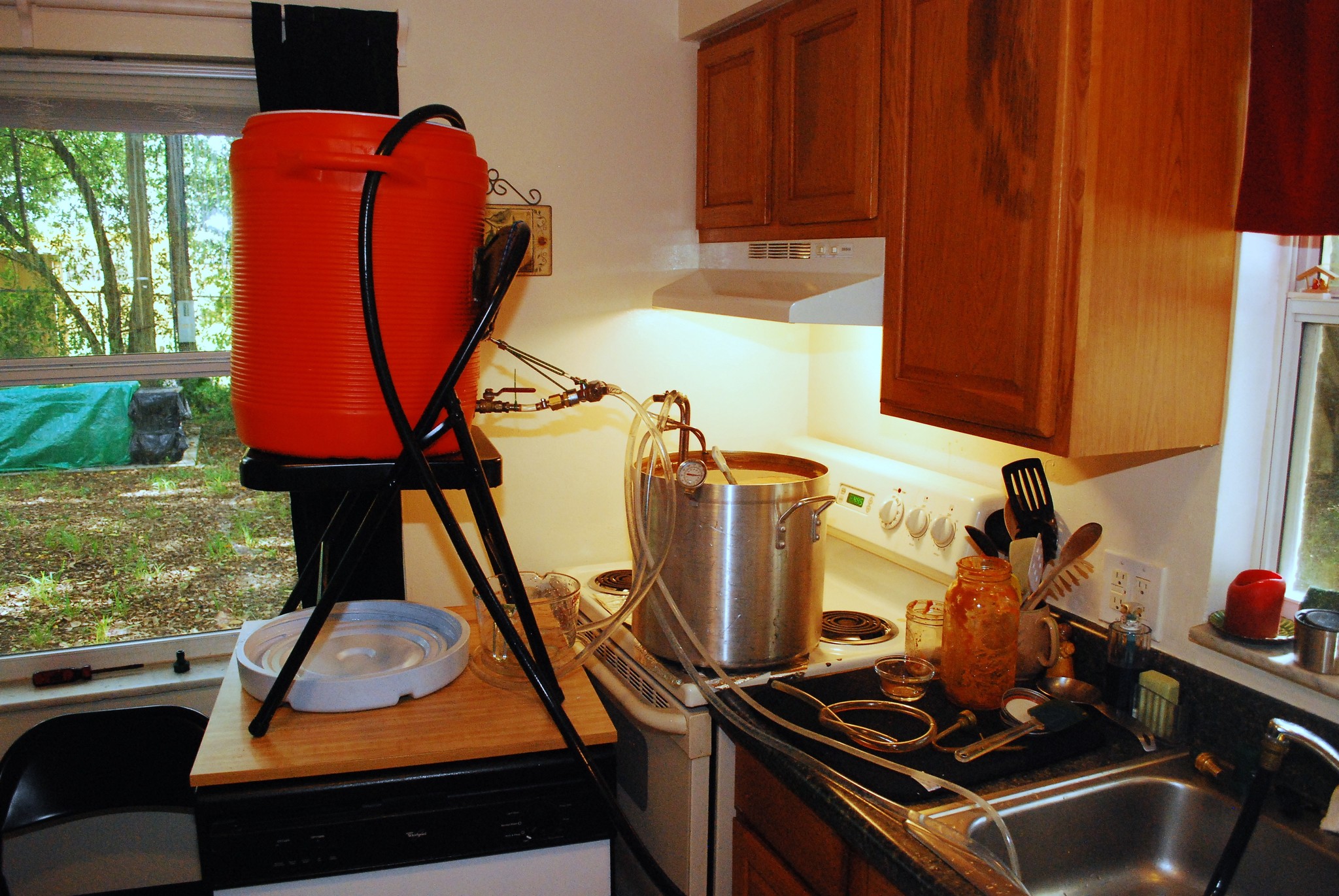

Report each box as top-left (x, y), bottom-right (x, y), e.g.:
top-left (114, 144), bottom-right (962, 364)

top-left (693, 0), bottom-right (890, 247)
top-left (727, 745), bottom-right (900, 896)
top-left (879, 0), bottom-right (1245, 458)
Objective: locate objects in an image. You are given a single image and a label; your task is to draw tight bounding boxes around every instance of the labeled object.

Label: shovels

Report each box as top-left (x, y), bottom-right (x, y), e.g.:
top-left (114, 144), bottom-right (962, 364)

top-left (964, 458), bottom-right (1103, 610)
top-left (954, 697), bottom-right (1089, 762)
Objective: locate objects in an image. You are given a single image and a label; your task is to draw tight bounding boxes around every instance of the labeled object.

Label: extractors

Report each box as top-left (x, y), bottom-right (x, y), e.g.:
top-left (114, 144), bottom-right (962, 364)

top-left (652, 236), bottom-right (884, 330)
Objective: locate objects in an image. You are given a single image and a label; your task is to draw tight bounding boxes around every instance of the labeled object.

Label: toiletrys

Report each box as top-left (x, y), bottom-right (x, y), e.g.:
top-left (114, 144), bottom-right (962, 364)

top-left (1105, 602), bottom-right (1152, 721)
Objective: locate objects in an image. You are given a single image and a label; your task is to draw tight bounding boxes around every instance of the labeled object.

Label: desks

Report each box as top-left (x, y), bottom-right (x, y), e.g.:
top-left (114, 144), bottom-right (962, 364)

top-left (187, 593), bottom-right (618, 896)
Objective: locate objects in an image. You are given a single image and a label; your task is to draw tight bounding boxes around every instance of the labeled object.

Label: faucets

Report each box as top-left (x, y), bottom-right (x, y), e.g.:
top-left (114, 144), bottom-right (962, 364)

top-left (1265, 718), bottom-right (1339, 777)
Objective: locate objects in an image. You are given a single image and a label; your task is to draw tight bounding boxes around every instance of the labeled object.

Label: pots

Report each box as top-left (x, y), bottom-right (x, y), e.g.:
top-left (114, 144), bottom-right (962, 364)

top-left (635, 450), bottom-right (838, 672)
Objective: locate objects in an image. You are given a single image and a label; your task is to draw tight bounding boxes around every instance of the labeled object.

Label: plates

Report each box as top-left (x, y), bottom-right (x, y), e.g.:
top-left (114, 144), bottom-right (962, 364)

top-left (1207, 609), bottom-right (1296, 644)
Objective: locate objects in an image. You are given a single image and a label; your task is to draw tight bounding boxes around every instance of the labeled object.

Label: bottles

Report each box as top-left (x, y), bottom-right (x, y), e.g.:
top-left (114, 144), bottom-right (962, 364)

top-left (941, 556), bottom-right (1020, 713)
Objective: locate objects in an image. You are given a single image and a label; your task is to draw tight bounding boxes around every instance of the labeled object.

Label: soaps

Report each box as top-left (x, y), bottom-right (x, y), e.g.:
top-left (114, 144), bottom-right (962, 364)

top-left (1135, 669), bottom-right (1181, 739)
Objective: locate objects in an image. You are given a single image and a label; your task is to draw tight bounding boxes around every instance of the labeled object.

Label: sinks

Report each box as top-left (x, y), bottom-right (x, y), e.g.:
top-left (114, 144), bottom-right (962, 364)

top-left (902, 746), bottom-right (1339, 896)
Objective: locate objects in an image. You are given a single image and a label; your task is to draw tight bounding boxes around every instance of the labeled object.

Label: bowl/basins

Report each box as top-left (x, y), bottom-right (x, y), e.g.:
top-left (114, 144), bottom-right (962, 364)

top-left (875, 655), bottom-right (936, 702)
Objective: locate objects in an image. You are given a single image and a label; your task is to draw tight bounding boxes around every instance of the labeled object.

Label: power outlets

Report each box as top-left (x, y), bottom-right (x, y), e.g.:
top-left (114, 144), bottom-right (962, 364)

top-left (1099, 549), bottom-right (1167, 642)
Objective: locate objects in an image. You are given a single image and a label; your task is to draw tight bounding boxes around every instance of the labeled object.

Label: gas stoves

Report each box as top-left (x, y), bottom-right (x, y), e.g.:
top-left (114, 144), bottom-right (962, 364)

top-left (550, 437), bottom-right (1009, 707)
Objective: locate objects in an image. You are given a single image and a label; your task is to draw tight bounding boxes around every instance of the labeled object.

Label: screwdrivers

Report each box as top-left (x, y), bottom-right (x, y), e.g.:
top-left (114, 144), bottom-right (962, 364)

top-left (32, 663), bottom-right (144, 688)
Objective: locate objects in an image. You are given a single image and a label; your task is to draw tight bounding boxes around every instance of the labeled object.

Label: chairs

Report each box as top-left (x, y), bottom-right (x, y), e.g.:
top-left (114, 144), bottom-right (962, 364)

top-left (2, 704), bottom-right (213, 895)
top-left (235, 215), bottom-right (571, 738)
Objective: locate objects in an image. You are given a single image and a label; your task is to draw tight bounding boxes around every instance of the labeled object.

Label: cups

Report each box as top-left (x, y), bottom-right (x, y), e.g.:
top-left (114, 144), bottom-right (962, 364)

top-left (472, 572), bottom-right (581, 677)
top-left (905, 599), bottom-right (945, 681)
top-left (1021, 599), bottom-right (1060, 679)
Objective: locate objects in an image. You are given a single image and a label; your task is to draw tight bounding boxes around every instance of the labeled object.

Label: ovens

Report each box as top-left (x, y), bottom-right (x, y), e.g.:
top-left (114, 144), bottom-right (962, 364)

top-left (570, 610), bottom-right (736, 896)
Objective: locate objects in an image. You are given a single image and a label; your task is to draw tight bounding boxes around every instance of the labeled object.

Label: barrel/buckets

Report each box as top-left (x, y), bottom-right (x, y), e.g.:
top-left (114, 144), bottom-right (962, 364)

top-left (227, 108), bottom-right (488, 459)
